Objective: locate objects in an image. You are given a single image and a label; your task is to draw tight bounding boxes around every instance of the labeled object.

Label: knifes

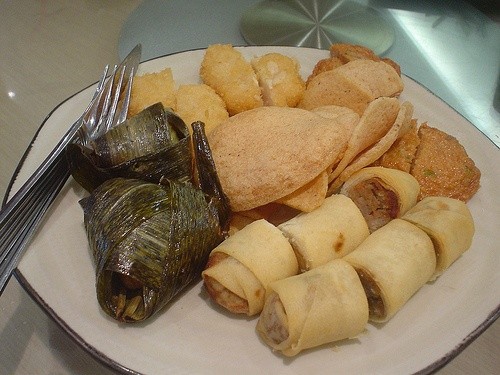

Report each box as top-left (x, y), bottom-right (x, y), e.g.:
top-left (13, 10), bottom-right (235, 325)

top-left (0, 43), bottom-right (143, 232)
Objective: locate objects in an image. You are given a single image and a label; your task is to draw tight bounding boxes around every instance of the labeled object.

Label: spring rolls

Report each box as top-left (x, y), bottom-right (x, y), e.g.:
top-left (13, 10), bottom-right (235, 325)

top-left (202, 167), bottom-right (475, 357)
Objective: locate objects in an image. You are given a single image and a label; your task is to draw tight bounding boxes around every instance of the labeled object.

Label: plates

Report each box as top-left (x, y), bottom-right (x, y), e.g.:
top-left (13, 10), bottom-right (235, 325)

top-left (2, 42), bottom-right (500, 375)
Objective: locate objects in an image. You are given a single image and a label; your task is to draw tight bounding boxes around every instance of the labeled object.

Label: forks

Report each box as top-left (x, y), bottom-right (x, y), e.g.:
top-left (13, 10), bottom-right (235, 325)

top-left (0, 61), bottom-right (135, 298)
